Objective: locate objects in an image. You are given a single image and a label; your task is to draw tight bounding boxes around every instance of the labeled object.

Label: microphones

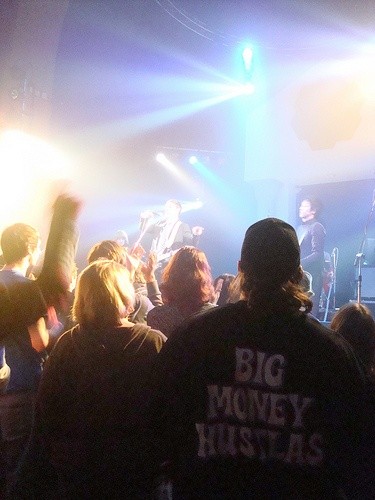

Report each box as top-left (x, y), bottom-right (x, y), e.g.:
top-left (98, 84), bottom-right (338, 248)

top-left (153, 210), bottom-right (165, 214)
top-left (331, 248), bottom-right (335, 258)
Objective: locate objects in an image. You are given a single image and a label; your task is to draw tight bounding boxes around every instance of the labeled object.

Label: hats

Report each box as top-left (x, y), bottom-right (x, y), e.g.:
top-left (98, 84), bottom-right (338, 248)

top-left (241, 218), bottom-right (301, 273)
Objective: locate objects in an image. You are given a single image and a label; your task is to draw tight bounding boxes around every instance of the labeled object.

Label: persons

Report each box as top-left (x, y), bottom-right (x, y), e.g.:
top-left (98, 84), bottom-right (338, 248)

top-left (9, 260), bottom-right (167, 500)
top-left (146, 218), bottom-right (369, 500)
top-left (211, 273), bottom-right (242, 305)
top-left (145, 248), bottom-right (217, 334)
top-left (88, 228), bottom-right (165, 325)
top-left (0, 224), bottom-right (48, 500)
top-left (296, 195), bottom-right (326, 317)
top-left (330, 301), bottom-right (375, 368)
top-left (140, 199), bottom-right (204, 286)
